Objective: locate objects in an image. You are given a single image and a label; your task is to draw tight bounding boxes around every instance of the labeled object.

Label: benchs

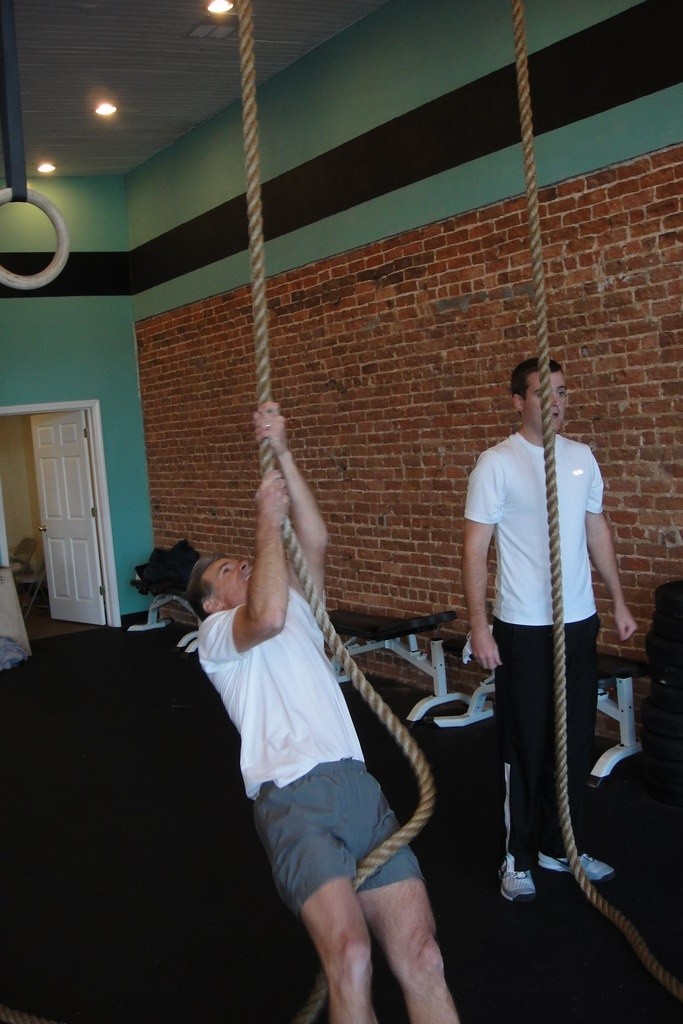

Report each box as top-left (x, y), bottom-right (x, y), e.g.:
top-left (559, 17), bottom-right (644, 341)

top-left (123, 572), bottom-right (651, 803)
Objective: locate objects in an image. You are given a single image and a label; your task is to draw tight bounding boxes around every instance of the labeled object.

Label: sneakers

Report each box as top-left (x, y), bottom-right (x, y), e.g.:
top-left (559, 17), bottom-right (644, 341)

top-left (498, 859), bottom-right (535, 901)
top-left (538, 851), bottom-right (614, 884)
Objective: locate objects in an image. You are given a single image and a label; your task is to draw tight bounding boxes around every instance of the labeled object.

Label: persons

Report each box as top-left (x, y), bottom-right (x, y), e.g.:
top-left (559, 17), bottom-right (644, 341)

top-left (463, 358), bottom-right (635, 900)
top-left (187, 401), bottom-right (462, 1024)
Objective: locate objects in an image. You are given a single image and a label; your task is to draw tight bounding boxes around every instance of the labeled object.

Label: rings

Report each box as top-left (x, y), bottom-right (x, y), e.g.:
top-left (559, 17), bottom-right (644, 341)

top-left (265, 421), bottom-right (271, 430)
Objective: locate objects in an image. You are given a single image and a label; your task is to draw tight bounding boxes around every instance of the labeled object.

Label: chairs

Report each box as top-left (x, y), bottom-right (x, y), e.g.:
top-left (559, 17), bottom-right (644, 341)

top-left (8, 537), bottom-right (52, 621)
